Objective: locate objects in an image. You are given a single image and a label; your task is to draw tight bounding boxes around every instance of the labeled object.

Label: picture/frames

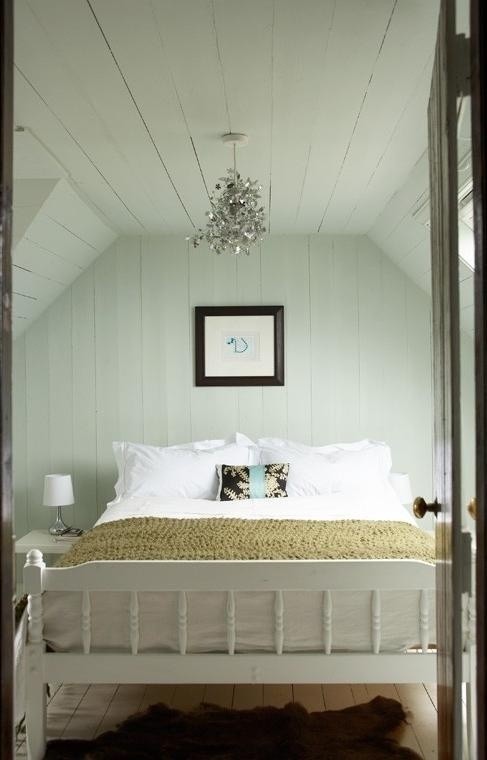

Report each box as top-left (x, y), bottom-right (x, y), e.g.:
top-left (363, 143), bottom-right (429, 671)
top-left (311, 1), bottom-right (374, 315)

top-left (192, 304), bottom-right (285, 388)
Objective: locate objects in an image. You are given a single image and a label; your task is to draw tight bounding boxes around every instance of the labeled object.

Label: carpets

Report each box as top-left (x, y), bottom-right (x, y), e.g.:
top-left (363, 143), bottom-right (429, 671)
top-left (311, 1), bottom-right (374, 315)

top-left (46, 693), bottom-right (422, 760)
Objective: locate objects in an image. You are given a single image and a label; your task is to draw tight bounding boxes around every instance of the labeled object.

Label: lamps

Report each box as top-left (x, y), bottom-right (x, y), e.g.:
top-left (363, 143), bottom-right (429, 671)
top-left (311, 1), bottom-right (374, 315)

top-left (186, 133), bottom-right (267, 257)
top-left (40, 473), bottom-right (75, 536)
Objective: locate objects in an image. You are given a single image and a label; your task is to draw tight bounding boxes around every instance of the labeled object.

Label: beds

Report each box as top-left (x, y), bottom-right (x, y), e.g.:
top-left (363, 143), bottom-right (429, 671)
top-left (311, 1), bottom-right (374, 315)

top-left (9, 428), bottom-right (470, 760)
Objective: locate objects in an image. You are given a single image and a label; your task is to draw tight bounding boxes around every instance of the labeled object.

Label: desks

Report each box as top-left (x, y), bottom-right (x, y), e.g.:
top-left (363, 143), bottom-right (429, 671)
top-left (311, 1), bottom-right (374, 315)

top-left (14, 530), bottom-right (81, 556)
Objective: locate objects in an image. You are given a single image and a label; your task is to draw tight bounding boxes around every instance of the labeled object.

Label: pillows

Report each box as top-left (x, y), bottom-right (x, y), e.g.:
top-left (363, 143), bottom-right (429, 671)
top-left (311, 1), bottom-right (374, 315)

top-left (109, 438), bottom-right (253, 505)
top-left (258, 438), bottom-right (395, 508)
top-left (212, 460), bottom-right (291, 505)
